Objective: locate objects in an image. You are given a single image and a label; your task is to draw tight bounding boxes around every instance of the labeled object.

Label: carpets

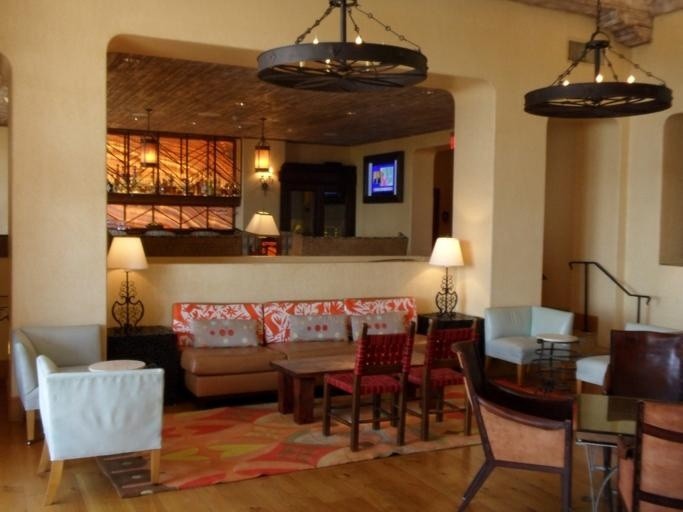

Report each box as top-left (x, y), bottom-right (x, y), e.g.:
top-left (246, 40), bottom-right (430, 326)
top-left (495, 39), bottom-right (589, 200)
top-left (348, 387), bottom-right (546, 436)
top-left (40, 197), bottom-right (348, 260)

top-left (93, 382), bottom-right (482, 499)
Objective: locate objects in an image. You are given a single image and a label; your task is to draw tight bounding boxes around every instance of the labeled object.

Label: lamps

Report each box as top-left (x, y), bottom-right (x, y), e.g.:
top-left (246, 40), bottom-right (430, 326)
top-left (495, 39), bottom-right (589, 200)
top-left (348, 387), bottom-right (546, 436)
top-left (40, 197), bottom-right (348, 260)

top-left (429, 237), bottom-right (465, 321)
top-left (523, 0), bottom-right (672, 119)
top-left (244, 208), bottom-right (280, 255)
top-left (140, 108), bottom-right (159, 166)
top-left (254, 118), bottom-right (272, 169)
top-left (260, 175), bottom-right (271, 189)
top-left (106, 236), bottom-right (148, 336)
top-left (256, 0), bottom-right (428, 93)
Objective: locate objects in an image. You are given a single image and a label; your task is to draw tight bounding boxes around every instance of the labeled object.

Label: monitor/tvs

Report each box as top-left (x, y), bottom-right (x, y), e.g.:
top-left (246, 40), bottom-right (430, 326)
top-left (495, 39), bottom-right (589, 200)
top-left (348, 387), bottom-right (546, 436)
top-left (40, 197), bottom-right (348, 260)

top-left (363, 151), bottom-right (403, 203)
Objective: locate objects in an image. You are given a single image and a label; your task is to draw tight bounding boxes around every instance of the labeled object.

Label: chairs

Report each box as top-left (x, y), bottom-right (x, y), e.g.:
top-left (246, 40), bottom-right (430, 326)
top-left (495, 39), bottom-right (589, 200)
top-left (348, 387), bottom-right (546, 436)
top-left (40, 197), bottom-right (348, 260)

top-left (607, 328), bottom-right (683, 511)
top-left (391, 328), bottom-right (479, 442)
top-left (483, 305), bottom-right (576, 386)
top-left (10, 324), bottom-right (102, 446)
top-left (449, 336), bottom-right (579, 511)
top-left (323, 321), bottom-right (415, 452)
top-left (36, 353), bottom-right (166, 506)
top-left (576, 323), bottom-right (683, 395)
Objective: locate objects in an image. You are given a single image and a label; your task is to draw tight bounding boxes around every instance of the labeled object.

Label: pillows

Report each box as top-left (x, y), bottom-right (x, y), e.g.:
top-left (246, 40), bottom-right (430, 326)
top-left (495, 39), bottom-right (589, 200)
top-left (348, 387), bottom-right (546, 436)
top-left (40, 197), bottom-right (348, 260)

top-left (172, 295), bottom-right (418, 348)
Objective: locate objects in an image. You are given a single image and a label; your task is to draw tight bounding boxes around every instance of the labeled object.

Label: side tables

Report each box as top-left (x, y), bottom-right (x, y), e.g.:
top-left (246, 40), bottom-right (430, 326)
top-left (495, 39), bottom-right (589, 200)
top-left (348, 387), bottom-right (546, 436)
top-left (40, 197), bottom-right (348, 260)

top-left (87, 359), bottom-right (146, 372)
top-left (107, 325), bottom-right (181, 404)
top-left (417, 311), bottom-right (485, 337)
top-left (531, 333), bottom-right (580, 393)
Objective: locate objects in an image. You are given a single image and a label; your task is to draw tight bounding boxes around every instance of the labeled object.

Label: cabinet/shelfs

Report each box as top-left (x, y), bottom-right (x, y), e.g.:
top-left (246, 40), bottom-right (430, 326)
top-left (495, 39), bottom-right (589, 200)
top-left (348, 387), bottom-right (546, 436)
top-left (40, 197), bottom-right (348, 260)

top-left (279, 161), bottom-right (357, 236)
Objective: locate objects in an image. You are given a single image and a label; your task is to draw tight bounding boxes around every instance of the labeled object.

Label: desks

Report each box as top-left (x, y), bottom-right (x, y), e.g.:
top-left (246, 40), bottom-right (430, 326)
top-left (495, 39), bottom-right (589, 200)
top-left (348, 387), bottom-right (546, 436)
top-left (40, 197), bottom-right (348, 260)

top-left (577, 393), bottom-right (637, 512)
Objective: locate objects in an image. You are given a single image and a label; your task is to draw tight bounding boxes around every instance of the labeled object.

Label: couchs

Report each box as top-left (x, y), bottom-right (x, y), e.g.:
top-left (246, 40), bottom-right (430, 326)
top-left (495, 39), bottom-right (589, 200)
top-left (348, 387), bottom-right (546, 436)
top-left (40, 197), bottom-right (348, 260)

top-left (172, 296), bottom-right (428, 410)
top-left (279, 231), bottom-right (408, 256)
top-left (107, 229), bottom-right (243, 257)
